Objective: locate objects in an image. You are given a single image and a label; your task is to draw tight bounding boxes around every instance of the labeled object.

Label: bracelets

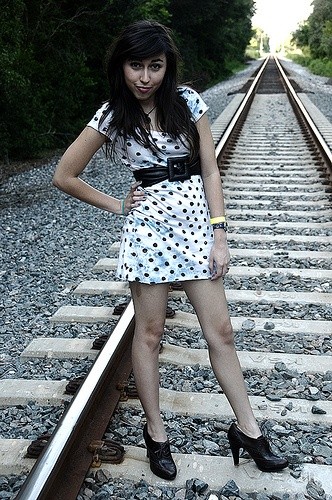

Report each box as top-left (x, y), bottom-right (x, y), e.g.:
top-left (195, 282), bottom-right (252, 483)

top-left (210, 216), bottom-right (227, 224)
top-left (120, 198), bottom-right (125, 213)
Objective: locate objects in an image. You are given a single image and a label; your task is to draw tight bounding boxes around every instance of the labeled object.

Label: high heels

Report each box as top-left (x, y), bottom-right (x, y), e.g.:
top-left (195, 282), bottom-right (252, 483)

top-left (143, 423), bottom-right (177, 480)
top-left (227, 422), bottom-right (289, 472)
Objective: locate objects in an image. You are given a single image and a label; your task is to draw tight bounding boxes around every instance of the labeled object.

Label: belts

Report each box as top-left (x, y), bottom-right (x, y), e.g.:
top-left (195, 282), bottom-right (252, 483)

top-left (132, 156), bottom-right (202, 188)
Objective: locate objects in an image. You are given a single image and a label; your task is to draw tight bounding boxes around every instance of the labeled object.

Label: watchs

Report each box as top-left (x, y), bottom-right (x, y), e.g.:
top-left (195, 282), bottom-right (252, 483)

top-left (213, 222), bottom-right (229, 232)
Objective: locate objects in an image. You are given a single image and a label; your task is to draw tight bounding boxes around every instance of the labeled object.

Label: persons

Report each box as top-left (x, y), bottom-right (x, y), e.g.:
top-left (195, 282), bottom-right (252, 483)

top-left (53, 21), bottom-right (288, 480)
